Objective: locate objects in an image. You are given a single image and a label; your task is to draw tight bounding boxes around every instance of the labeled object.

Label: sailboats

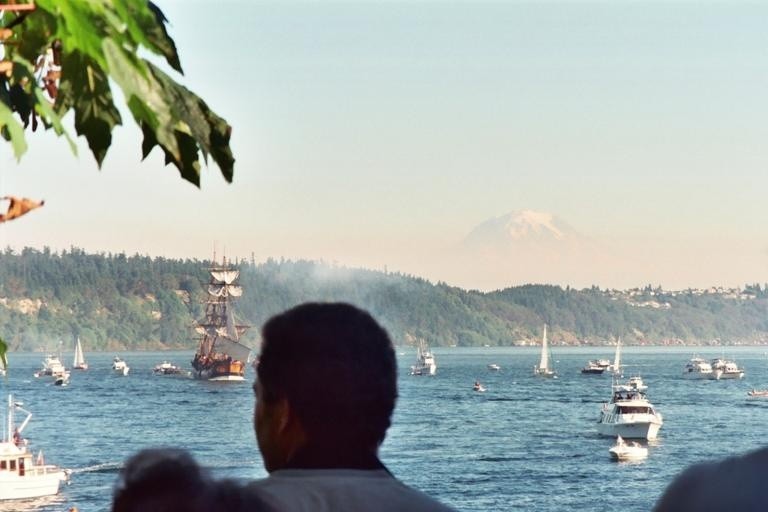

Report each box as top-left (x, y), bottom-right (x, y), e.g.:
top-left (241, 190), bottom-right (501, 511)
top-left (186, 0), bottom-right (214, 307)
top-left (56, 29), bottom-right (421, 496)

top-left (533, 325), bottom-right (557, 379)
top-left (190, 252), bottom-right (252, 382)
top-left (73, 337), bottom-right (87, 370)
top-left (608, 336), bottom-right (625, 380)
top-left (410, 338), bottom-right (436, 375)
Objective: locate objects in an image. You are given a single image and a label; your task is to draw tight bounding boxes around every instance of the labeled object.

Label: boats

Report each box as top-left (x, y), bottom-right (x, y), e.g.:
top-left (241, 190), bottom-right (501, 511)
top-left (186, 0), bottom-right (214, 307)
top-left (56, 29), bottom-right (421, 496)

top-left (111, 355), bottom-right (129, 376)
top-left (40, 355), bottom-right (71, 387)
top-left (679, 357), bottom-right (744, 379)
top-left (0, 394), bottom-right (68, 501)
top-left (152, 364), bottom-right (180, 376)
top-left (608, 436), bottom-right (647, 461)
top-left (624, 372), bottom-right (647, 393)
top-left (743, 388), bottom-right (767, 408)
top-left (591, 386), bottom-right (662, 442)
top-left (581, 359), bottom-right (609, 373)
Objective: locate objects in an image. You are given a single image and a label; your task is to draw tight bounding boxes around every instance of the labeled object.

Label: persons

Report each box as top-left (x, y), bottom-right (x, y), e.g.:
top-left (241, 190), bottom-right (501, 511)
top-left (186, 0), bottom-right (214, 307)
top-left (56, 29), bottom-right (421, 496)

top-left (247, 301), bottom-right (453, 512)
top-left (14, 426), bottom-right (20, 445)
